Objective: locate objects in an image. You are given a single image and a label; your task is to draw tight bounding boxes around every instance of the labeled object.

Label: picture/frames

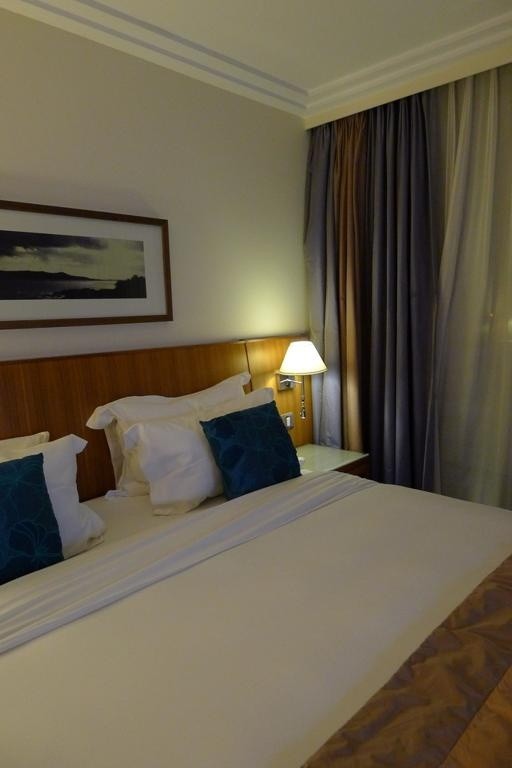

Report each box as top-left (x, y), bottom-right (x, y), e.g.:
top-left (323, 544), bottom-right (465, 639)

top-left (0, 198), bottom-right (174, 332)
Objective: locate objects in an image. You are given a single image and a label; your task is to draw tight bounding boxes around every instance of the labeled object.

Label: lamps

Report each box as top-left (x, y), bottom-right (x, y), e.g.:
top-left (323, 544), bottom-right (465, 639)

top-left (276, 335), bottom-right (327, 422)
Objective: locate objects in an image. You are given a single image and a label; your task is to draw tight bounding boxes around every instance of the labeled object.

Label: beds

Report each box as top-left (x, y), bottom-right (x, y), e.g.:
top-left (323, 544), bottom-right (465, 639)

top-left (0, 334), bottom-right (512, 766)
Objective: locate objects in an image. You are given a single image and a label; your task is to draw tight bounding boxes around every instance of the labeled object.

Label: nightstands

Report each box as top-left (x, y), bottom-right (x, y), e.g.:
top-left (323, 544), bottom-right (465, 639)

top-left (295, 443), bottom-right (369, 479)
